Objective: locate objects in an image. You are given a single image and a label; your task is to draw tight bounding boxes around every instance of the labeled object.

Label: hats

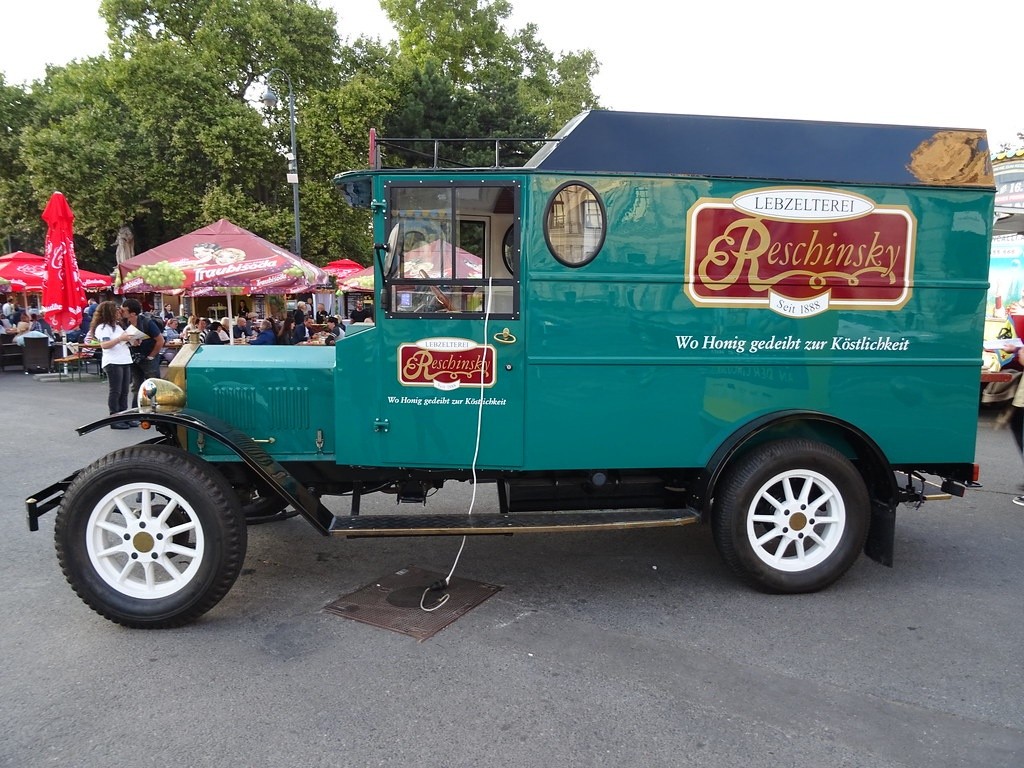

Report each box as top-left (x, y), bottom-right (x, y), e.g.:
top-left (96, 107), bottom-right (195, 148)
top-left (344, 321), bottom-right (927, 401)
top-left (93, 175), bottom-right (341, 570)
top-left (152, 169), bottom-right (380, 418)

top-left (327, 317), bottom-right (338, 324)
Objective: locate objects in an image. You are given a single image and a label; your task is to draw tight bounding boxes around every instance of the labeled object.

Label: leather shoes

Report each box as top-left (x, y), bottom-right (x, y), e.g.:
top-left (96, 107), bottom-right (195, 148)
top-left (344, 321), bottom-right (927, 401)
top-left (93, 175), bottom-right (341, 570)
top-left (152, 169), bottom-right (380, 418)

top-left (111, 421), bottom-right (138, 429)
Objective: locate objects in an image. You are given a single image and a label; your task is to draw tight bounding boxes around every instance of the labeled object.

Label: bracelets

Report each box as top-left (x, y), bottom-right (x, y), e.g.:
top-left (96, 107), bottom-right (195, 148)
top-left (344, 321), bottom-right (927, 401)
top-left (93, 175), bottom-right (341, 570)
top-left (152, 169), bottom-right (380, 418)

top-left (147, 356), bottom-right (154, 360)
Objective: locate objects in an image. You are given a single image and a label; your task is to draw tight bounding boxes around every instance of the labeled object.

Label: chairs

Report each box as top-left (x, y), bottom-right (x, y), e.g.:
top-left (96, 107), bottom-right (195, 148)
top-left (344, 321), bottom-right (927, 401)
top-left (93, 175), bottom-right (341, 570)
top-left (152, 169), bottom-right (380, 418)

top-left (0, 333), bottom-right (260, 381)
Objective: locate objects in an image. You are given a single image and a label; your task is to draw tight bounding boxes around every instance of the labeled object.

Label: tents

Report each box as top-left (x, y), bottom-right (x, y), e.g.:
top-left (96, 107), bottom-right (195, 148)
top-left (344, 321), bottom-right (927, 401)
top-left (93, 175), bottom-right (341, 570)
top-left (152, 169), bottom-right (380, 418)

top-left (114, 219), bottom-right (327, 345)
top-left (0, 250), bottom-right (115, 313)
top-left (320, 239), bottom-right (482, 319)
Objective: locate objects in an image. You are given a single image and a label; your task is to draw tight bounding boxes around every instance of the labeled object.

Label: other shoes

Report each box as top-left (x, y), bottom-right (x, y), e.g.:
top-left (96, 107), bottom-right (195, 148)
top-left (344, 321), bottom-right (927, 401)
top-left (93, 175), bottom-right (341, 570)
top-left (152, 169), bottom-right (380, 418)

top-left (1012, 495), bottom-right (1024, 505)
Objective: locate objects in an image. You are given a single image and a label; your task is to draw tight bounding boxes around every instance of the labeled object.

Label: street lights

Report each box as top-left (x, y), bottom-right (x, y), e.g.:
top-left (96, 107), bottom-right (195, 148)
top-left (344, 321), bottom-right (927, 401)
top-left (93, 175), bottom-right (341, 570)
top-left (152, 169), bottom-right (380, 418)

top-left (259, 68), bottom-right (303, 255)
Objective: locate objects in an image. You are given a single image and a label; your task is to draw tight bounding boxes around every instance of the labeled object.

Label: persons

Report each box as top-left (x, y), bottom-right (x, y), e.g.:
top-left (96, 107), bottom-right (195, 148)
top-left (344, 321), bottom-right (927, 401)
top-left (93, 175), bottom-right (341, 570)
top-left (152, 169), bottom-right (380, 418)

top-left (90, 299), bottom-right (165, 430)
top-left (0, 293), bottom-right (56, 347)
top-left (80, 298), bottom-right (98, 333)
top-left (164, 297), bottom-right (372, 346)
top-left (985, 343), bottom-right (1024, 506)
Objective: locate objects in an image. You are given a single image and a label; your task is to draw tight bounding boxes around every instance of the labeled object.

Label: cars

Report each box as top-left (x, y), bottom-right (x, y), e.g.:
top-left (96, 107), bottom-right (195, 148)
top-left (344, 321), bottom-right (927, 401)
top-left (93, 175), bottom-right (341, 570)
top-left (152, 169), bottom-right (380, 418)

top-left (19, 110), bottom-right (998, 631)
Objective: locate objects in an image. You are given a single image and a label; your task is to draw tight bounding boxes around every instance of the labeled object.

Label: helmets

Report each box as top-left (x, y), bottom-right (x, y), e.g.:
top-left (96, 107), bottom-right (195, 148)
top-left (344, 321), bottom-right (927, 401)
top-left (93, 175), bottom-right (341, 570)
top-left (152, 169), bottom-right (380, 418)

top-left (248, 312), bottom-right (259, 318)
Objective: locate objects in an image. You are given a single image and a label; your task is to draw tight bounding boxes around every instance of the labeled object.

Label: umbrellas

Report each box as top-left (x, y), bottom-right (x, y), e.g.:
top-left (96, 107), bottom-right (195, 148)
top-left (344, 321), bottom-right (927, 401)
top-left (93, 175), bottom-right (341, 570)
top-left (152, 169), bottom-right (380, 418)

top-left (40, 192), bottom-right (88, 375)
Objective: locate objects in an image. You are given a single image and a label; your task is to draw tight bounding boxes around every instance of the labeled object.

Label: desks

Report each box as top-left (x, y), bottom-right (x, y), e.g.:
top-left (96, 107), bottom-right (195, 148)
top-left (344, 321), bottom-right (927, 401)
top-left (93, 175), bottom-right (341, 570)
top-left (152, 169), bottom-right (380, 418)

top-left (164, 340), bottom-right (183, 351)
top-left (224, 336), bottom-right (255, 345)
top-left (294, 331), bottom-right (332, 346)
top-left (208, 306), bottom-right (227, 320)
